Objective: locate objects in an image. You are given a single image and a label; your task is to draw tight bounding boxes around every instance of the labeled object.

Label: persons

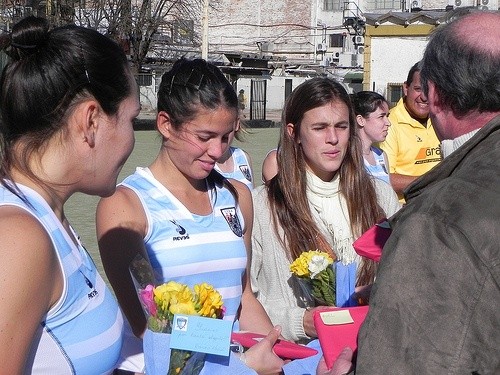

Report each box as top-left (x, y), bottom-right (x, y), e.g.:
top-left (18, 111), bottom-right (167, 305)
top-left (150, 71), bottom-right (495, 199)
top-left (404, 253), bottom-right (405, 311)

top-left (213, 112), bottom-right (254, 192)
top-left (261, 147), bottom-right (279, 184)
top-left (379, 61), bottom-right (443, 204)
top-left (95, 57), bottom-right (306, 375)
top-left (249, 77), bottom-right (402, 347)
top-left (0, 14), bottom-right (142, 375)
top-left (349, 91), bottom-right (403, 219)
top-left (315, 9), bottom-right (500, 375)
top-left (237, 88), bottom-right (247, 120)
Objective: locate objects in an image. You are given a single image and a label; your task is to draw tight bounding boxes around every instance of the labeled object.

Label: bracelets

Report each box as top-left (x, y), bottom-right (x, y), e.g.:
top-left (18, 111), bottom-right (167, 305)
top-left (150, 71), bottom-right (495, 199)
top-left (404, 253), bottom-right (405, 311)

top-left (239, 351), bottom-right (247, 364)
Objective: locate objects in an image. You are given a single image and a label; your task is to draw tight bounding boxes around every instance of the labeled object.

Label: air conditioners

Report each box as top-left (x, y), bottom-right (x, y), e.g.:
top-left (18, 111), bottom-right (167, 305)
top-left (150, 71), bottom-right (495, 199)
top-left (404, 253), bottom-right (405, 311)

top-left (333, 52), bottom-right (340, 59)
top-left (454, 0), bottom-right (471, 8)
top-left (481, 0), bottom-right (498, 10)
top-left (316, 43), bottom-right (326, 51)
top-left (355, 36), bottom-right (364, 44)
top-left (406, 0), bottom-right (422, 10)
top-left (358, 46), bottom-right (364, 54)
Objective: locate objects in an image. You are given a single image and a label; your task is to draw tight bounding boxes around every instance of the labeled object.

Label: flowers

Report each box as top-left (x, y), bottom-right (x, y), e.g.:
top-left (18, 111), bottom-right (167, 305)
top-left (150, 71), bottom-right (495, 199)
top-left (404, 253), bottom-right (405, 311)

top-left (289, 249), bottom-right (337, 306)
top-left (137, 281), bottom-right (226, 374)
